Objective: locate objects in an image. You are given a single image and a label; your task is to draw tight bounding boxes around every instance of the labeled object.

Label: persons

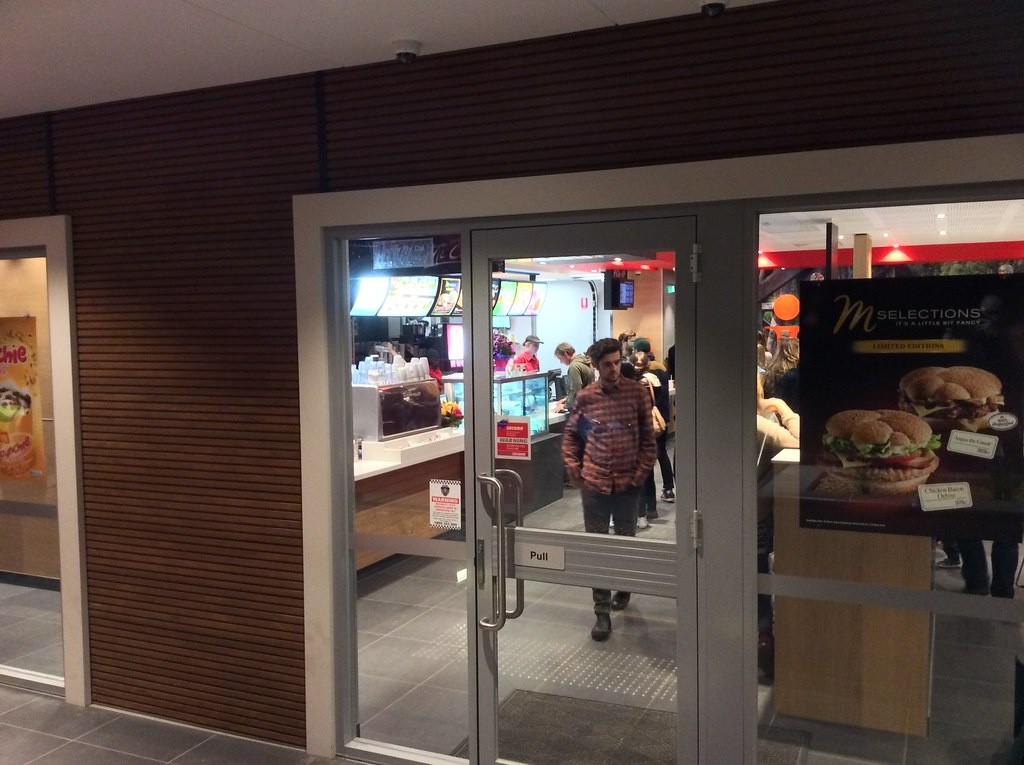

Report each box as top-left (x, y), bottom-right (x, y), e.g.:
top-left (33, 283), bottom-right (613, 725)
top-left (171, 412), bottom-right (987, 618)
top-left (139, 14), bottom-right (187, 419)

top-left (387, 342), bottom-right (444, 392)
top-left (562, 330), bottom-right (676, 641)
top-left (504, 335), bottom-right (545, 372)
top-left (934, 538), bottom-right (1019, 600)
top-left (752, 311), bottom-right (800, 680)
top-left (554, 342), bottom-right (596, 440)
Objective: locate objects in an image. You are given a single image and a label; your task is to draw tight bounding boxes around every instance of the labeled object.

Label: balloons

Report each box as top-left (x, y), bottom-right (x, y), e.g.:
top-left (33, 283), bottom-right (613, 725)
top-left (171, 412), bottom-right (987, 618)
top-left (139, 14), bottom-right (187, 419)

top-left (774, 294), bottom-right (800, 320)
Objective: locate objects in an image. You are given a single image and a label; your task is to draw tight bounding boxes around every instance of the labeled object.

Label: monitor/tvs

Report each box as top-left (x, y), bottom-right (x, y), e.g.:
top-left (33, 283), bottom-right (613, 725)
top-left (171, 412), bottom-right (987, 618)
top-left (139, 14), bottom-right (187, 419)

top-left (548, 368), bottom-right (562, 386)
top-left (617, 279), bottom-right (634, 308)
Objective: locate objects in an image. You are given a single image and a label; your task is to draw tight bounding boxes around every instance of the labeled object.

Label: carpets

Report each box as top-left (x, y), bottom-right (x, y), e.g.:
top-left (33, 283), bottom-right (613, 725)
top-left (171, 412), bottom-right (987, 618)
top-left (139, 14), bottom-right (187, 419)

top-left (451, 689), bottom-right (812, 765)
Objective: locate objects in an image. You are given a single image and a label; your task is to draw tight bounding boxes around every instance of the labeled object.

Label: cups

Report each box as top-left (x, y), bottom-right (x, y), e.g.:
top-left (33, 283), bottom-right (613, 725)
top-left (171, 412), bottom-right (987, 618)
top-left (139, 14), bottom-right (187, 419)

top-left (351, 357), bottom-right (393, 387)
top-left (392, 355), bottom-right (429, 383)
top-left (506, 363), bottom-right (526, 378)
top-left (668, 380), bottom-right (674, 390)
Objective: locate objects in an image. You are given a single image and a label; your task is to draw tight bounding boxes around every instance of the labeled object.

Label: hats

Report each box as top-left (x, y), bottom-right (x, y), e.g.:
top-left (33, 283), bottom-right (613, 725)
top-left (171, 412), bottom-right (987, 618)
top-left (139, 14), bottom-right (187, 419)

top-left (421, 350), bottom-right (439, 358)
top-left (525, 335), bottom-right (544, 344)
top-left (628, 337), bottom-right (651, 353)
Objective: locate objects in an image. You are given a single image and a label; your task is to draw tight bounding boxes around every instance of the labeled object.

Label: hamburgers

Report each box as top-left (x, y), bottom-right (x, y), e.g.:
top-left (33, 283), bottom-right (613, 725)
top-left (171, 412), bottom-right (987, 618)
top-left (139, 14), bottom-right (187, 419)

top-left (820, 408), bottom-right (942, 496)
top-left (897, 365), bottom-right (1005, 433)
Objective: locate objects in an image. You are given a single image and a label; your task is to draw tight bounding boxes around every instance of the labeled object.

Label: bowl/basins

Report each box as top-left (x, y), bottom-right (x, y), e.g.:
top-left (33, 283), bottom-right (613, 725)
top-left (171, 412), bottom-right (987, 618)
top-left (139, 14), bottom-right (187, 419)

top-left (412, 324), bottom-right (425, 336)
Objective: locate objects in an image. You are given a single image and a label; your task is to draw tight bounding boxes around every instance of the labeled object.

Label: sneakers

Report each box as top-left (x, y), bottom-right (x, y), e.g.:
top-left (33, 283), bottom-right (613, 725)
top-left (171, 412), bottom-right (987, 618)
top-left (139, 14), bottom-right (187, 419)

top-left (609, 515), bottom-right (614, 527)
top-left (637, 517), bottom-right (648, 529)
top-left (660, 489), bottom-right (675, 501)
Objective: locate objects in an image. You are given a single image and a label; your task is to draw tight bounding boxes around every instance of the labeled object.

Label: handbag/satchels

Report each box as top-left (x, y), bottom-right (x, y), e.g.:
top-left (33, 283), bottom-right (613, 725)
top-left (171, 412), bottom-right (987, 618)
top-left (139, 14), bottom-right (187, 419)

top-left (648, 380), bottom-right (666, 437)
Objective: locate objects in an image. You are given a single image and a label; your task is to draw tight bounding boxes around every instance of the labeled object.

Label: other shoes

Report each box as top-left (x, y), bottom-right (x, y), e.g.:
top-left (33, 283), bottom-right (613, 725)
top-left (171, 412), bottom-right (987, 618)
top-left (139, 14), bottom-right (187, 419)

top-left (591, 612), bottom-right (612, 642)
top-left (611, 591), bottom-right (631, 610)
top-left (937, 560), bottom-right (962, 568)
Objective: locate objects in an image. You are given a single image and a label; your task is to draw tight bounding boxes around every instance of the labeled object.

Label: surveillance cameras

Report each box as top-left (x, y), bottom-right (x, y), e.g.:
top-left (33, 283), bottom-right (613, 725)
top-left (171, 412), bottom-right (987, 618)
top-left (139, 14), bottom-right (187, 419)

top-left (392, 40), bottom-right (421, 66)
